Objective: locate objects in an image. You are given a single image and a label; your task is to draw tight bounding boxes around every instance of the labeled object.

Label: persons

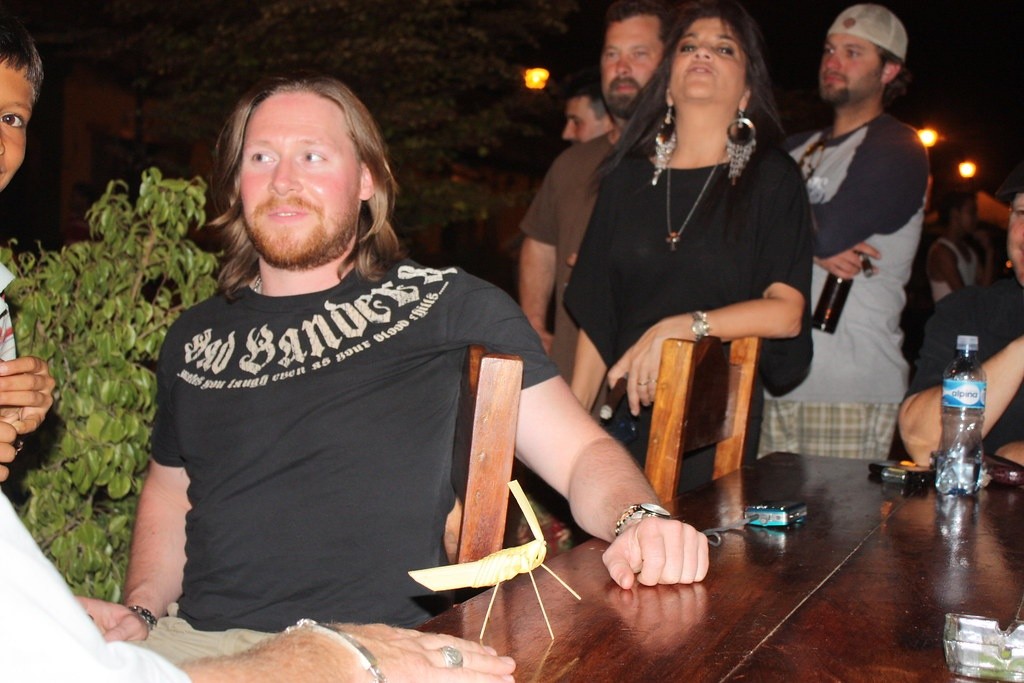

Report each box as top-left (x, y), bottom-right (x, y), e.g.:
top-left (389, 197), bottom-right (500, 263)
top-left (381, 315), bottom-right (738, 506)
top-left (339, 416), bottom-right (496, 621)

top-left (515, 0), bottom-right (1024, 495)
top-left (71, 73), bottom-right (709, 665)
top-left (0, 29), bottom-right (517, 683)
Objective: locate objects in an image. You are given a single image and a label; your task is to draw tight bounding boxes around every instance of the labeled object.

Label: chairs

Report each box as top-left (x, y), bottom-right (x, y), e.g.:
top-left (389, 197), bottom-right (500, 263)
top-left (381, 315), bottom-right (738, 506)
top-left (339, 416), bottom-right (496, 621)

top-left (455, 344), bottom-right (527, 571)
top-left (643, 329), bottom-right (762, 508)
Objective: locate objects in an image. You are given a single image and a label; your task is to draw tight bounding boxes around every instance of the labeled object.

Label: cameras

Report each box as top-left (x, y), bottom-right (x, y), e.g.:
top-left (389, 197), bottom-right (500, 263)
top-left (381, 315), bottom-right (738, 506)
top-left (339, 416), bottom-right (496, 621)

top-left (745, 501), bottom-right (807, 528)
top-left (868, 461), bottom-right (933, 485)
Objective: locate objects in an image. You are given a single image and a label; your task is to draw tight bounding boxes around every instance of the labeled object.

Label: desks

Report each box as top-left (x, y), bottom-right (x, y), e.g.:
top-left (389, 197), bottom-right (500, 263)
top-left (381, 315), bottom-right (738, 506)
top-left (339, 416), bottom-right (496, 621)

top-left (417, 449), bottom-right (1024, 683)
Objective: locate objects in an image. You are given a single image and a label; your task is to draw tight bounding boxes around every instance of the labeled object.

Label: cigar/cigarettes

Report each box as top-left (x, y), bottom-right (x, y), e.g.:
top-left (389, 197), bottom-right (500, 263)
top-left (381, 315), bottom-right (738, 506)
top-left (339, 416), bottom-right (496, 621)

top-left (599, 376), bottom-right (629, 420)
top-left (860, 253), bottom-right (873, 277)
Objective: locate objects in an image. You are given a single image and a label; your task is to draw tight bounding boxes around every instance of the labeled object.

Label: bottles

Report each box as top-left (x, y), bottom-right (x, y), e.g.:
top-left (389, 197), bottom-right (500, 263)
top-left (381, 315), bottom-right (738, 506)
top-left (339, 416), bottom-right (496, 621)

top-left (934, 335), bottom-right (985, 496)
top-left (812, 271), bottom-right (853, 334)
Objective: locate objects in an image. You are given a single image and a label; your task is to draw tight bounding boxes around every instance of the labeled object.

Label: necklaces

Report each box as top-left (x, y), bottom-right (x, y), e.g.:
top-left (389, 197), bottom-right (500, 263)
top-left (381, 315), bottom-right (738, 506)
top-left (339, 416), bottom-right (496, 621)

top-left (666, 149), bottom-right (726, 252)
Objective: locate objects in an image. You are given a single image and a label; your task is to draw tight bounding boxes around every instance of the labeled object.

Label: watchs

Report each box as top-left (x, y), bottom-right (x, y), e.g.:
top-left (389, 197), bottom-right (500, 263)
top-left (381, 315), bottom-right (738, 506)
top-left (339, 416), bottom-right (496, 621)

top-left (690, 310), bottom-right (709, 339)
top-left (614, 503), bottom-right (673, 536)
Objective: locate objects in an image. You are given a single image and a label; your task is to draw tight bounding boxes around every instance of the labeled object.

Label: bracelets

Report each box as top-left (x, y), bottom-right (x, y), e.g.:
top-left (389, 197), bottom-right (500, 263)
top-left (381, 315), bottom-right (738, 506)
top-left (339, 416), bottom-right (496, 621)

top-left (128, 605), bottom-right (158, 629)
top-left (284, 618), bottom-right (387, 683)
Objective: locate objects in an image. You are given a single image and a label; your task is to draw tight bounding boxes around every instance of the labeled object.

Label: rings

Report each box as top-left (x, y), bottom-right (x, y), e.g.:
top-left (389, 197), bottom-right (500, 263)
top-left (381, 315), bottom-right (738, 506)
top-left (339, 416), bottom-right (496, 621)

top-left (648, 380), bottom-right (656, 383)
top-left (637, 380), bottom-right (648, 386)
top-left (439, 645), bottom-right (464, 668)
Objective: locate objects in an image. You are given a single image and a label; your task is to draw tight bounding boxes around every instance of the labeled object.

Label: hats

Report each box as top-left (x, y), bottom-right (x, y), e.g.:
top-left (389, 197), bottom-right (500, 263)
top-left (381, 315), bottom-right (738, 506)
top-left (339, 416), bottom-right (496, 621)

top-left (827, 3), bottom-right (908, 61)
